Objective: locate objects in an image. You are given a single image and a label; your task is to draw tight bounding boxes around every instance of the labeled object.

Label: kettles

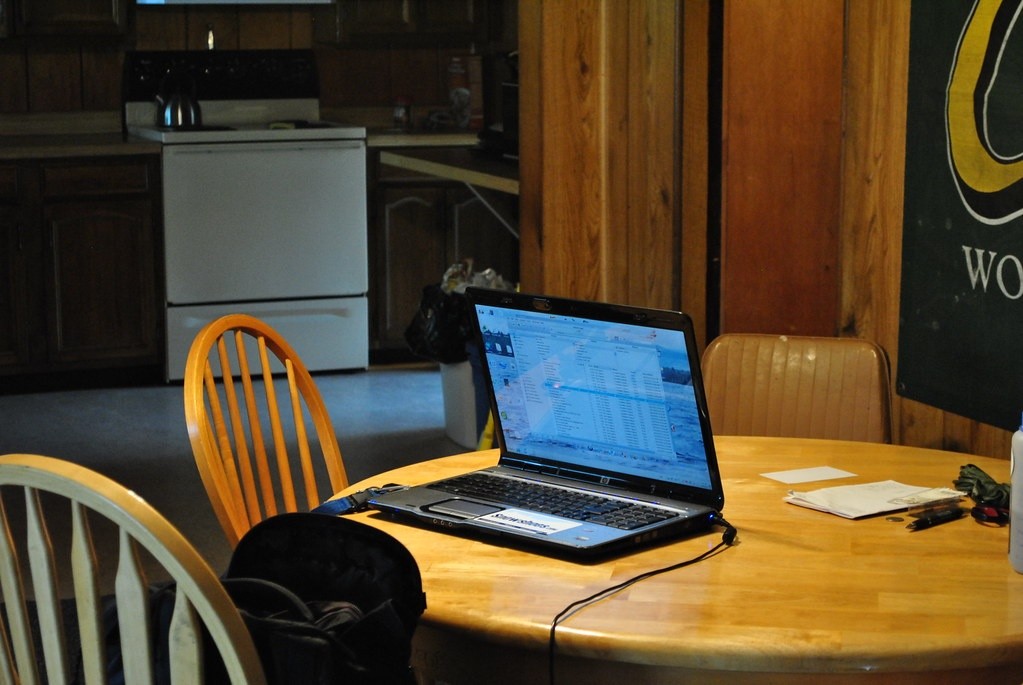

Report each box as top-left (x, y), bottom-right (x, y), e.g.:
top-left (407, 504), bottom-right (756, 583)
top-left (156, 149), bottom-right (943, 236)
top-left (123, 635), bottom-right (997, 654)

top-left (153, 72), bottom-right (201, 127)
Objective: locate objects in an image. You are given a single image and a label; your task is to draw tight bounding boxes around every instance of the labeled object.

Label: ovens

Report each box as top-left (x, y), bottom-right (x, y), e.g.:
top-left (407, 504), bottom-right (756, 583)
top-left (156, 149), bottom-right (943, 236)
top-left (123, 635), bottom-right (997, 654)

top-left (160, 145), bottom-right (370, 381)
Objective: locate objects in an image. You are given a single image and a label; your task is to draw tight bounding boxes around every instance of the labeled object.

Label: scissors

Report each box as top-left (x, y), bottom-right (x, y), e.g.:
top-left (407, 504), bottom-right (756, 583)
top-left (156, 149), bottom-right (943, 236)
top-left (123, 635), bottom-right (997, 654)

top-left (969, 494), bottom-right (1011, 525)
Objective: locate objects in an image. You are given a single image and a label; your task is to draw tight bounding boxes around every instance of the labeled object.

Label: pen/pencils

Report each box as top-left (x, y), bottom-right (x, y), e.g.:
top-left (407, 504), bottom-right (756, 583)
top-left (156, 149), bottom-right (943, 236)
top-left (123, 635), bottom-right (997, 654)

top-left (905, 507), bottom-right (969, 531)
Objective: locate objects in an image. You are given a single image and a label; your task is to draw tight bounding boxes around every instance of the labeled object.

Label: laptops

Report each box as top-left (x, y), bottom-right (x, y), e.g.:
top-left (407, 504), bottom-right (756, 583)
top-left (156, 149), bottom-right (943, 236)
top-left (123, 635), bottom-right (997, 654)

top-left (365, 286), bottom-right (726, 567)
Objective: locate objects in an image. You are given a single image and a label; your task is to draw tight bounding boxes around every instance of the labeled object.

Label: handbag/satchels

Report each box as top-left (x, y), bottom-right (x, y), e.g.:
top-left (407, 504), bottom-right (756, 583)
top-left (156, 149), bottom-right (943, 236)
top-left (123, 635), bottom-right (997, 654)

top-left (78, 512), bottom-right (427, 685)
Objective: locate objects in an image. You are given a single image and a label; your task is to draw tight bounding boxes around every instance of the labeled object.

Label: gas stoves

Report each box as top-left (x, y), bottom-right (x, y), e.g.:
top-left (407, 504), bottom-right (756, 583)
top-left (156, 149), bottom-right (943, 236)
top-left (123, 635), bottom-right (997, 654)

top-left (122, 51), bottom-right (366, 143)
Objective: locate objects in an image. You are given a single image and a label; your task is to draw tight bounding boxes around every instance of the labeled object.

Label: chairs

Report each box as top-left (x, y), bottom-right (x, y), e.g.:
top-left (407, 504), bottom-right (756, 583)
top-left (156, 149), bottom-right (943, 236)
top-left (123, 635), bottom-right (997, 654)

top-left (701, 333), bottom-right (893, 445)
top-left (0, 454), bottom-right (268, 685)
top-left (183, 315), bottom-right (350, 549)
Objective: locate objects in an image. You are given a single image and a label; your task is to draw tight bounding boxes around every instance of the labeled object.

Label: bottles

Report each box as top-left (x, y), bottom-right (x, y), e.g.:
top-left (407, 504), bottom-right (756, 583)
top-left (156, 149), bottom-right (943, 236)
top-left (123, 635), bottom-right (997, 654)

top-left (393, 96), bottom-right (410, 128)
top-left (1009, 411), bottom-right (1023, 575)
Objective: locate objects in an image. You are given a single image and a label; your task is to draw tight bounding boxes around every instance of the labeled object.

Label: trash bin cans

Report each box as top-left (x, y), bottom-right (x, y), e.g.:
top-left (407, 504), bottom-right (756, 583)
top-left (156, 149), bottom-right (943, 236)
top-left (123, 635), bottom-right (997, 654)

top-left (437, 361), bottom-right (490, 449)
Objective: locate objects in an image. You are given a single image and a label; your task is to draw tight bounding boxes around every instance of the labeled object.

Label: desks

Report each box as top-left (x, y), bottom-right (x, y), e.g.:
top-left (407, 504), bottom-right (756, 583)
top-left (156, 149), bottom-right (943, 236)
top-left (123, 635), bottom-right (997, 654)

top-left (330, 436), bottom-right (1023, 685)
top-left (381, 146), bottom-right (520, 241)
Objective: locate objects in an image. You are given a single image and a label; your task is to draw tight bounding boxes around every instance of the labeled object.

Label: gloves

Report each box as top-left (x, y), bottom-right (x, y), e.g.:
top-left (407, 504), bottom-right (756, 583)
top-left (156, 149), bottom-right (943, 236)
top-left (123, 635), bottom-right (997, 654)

top-left (953, 464), bottom-right (1009, 507)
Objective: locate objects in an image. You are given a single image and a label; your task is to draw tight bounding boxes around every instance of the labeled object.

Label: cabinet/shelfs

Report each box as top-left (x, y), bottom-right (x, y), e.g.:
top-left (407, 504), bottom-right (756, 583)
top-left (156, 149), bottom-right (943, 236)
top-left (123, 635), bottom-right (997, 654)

top-left (332, 106), bottom-right (484, 365)
top-left (0, 0), bottom-right (130, 37)
top-left (0, 109), bottom-right (165, 397)
top-left (312, 0), bottom-right (473, 49)
